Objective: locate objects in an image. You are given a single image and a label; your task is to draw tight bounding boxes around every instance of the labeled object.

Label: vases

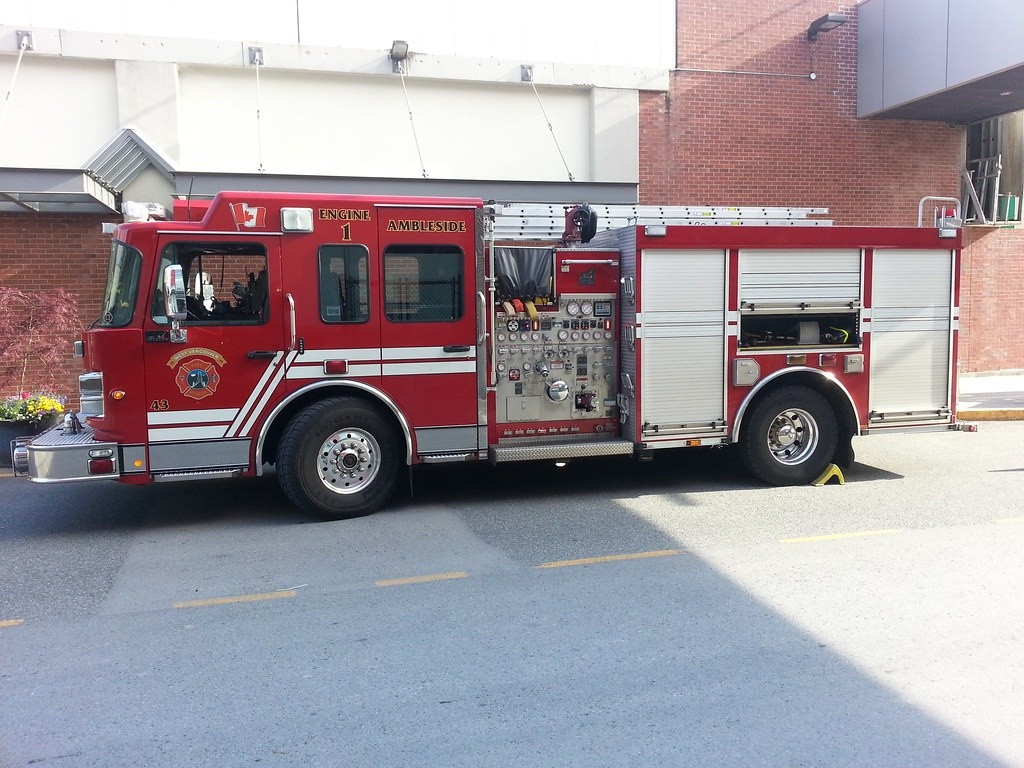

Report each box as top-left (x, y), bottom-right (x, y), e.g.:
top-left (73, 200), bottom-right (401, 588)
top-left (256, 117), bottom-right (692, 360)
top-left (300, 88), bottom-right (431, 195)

top-left (0, 417), bottom-right (58, 468)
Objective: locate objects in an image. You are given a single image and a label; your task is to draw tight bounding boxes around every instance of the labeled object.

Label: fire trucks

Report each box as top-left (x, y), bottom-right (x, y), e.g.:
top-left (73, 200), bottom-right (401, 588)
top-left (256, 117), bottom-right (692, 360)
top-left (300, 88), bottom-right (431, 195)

top-left (10, 188), bottom-right (983, 525)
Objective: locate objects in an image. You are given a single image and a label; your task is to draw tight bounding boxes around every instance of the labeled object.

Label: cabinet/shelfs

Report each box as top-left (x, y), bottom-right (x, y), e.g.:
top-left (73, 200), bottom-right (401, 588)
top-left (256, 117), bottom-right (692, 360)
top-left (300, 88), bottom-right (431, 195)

top-left (10, 436), bottom-right (36, 477)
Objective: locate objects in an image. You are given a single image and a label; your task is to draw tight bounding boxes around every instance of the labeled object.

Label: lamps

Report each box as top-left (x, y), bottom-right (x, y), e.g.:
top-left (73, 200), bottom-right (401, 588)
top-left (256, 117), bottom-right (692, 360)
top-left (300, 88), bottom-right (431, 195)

top-left (808, 14), bottom-right (847, 41)
top-left (390, 41), bottom-right (408, 74)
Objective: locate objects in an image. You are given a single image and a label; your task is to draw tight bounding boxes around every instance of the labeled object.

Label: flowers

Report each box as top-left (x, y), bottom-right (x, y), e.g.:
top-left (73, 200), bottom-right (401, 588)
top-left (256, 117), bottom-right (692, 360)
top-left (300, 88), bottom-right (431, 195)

top-left (0, 387), bottom-right (67, 429)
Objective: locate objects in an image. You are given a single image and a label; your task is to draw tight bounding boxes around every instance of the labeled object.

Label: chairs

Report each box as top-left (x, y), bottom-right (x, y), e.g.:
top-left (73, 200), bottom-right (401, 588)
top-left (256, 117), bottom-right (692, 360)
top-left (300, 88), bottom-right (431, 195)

top-left (247, 269), bottom-right (267, 313)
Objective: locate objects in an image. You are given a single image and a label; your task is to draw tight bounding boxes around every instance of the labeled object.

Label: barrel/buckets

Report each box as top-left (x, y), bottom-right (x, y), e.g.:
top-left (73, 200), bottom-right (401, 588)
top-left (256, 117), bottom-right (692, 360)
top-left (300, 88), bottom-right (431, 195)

top-left (1000, 192), bottom-right (1019, 221)
